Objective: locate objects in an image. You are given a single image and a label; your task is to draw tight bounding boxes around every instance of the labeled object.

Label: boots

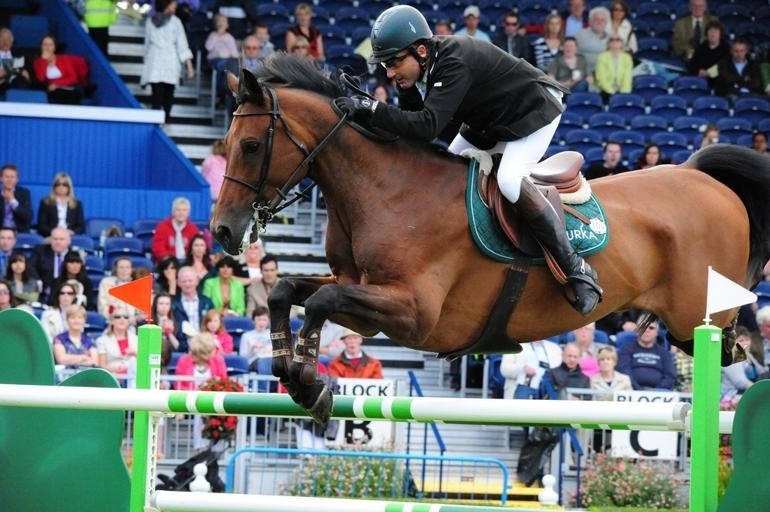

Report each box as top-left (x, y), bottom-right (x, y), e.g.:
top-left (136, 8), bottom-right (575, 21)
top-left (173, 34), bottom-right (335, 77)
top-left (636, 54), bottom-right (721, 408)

top-left (512, 176), bottom-right (599, 317)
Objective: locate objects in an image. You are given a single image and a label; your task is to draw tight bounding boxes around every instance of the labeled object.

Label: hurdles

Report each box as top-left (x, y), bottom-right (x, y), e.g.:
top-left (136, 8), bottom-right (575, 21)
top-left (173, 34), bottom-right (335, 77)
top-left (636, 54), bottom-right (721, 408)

top-left (129, 323), bottom-right (769, 512)
top-left (0, 321), bottom-right (722, 512)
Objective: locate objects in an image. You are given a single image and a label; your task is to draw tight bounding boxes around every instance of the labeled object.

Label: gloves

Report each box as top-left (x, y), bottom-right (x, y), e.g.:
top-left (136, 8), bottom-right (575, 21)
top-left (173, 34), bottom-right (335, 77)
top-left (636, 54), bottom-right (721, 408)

top-left (336, 94), bottom-right (377, 119)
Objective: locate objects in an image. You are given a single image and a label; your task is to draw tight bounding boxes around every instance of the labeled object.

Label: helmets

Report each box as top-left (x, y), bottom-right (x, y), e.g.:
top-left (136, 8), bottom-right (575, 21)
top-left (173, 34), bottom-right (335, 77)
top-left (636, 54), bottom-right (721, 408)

top-left (367, 5), bottom-right (433, 65)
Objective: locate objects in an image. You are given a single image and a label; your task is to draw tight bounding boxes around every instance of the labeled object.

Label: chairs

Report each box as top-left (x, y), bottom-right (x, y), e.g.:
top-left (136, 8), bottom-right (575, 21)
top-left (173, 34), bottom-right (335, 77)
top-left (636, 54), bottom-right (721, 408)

top-left (672, 76), bottom-right (708, 106)
top-left (630, 116), bottom-right (667, 144)
top-left (567, 92), bottom-right (603, 121)
top-left (629, 1), bottom-right (690, 59)
top-left (419, 0), bottom-right (609, 41)
top-left (85, 253), bottom-right (108, 273)
top-left (89, 278), bottom-right (103, 309)
top-left (73, 236), bottom-right (95, 254)
top-left (134, 219), bottom-right (161, 252)
top-left (757, 117), bottom-right (770, 140)
top-left (734, 98), bottom-right (770, 127)
top-left (565, 330), bottom-right (609, 344)
top-left (692, 98), bottom-right (730, 123)
top-left (254, 0), bottom-right (416, 66)
top-left (609, 131), bottom-right (646, 160)
top-left (257, 358), bottom-right (276, 392)
top-left (589, 115), bottom-right (627, 141)
top-left (15, 233), bottom-right (42, 257)
top-left (167, 352), bottom-right (184, 371)
top-left (104, 237), bottom-right (144, 269)
top-left (125, 257), bottom-right (153, 273)
top-left (224, 355), bottom-right (250, 374)
top-left (674, 151), bottom-right (693, 163)
top-left (566, 130), bottom-right (602, 159)
top-left (608, 94), bottom-right (644, 124)
top-left (754, 283), bottom-right (770, 307)
top-left (674, 117), bottom-right (708, 145)
top-left (585, 148), bottom-right (604, 163)
top-left (617, 332), bottom-right (667, 350)
top-left (693, 134), bottom-right (731, 151)
top-left (0, 15), bottom-right (94, 104)
top-left (222, 317), bottom-right (255, 352)
top-left (629, 150), bottom-right (665, 167)
top-left (555, 113), bottom-right (583, 140)
top-left (709, 0), bottom-right (770, 58)
top-left (716, 119), bottom-right (754, 144)
top-left (85, 313), bottom-right (106, 338)
top-left (548, 146), bottom-right (577, 157)
top-left (738, 134), bottom-right (753, 147)
top-left (651, 96), bottom-right (687, 125)
top-left (632, 76), bottom-right (667, 105)
top-left (86, 217), bottom-right (128, 237)
top-left (651, 133), bottom-right (687, 162)
top-left (488, 357), bottom-right (504, 396)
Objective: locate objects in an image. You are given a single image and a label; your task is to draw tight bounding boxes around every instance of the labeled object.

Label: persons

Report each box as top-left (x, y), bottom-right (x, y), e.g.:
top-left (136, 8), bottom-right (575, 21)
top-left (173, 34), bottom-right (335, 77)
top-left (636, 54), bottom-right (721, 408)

top-left (151, 196), bottom-right (201, 262)
top-left (501, 341), bottom-right (563, 399)
top-left (239, 306), bottom-right (272, 364)
top-left (687, 22), bottom-right (725, 82)
top-left (560, 0), bottom-right (585, 39)
top-left (752, 306), bottom-right (770, 365)
top-left (609, 1), bottom-right (639, 53)
top-left (47, 252), bottom-right (95, 309)
top-left (33, 35), bottom-right (86, 105)
top-left (576, 322), bottom-right (601, 374)
top-left (701, 125), bottom-right (720, 147)
top-left (583, 140), bottom-right (628, 179)
top-left (0, 165), bottom-right (34, 234)
top-left (247, 255), bottom-right (297, 324)
top-left (291, 35), bottom-right (311, 59)
top-left (254, 25), bottom-right (275, 58)
top-left (151, 292), bottom-right (187, 352)
top-left (0, 27), bottom-right (30, 103)
top-left (721, 327), bottom-right (766, 397)
top-left (335, 5), bottom-right (603, 318)
top-left (4, 252), bottom-right (38, 301)
top-left (31, 226), bottom-right (73, 302)
top-left (547, 36), bottom-right (594, 93)
top-left (618, 313), bottom-right (676, 390)
top-left (133, 267), bottom-right (153, 325)
top-left (97, 259), bottom-right (135, 324)
top-left (0, 227), bottom-right (17, 280)
top-left (238, 233), bottom-right (269, 281)
top-left (41, 282), bottom-right (76, 337)
top-left (533, 13), bottom-right (562, 71)
top-left (329, 329), bottom-right (383, 379)
top-left (671, 0), bottom-right (720, 58)
top-left (241, 36), bottom-right (264, 76)
top-left (752, 130), bottom-right (768, 156)
top-left (205, 14), bottom-right (240, 95)
top-left (453, 6), bottom-right (490, 42)
top-left (435, 20), bottom-right (452, 36)
top-left (0, 279), bottom-right (16, 310)
top-left (174, 332), bottom-right (226, 392)
top-left (202, 308), bottom-right (234, 354)
top-left (140, 1), bottom-right (195, 123)
top-left (492, 10), bottom-right (533, 62)
top-left (38, 171), bottom-right (83, 238)
top-left (286, 4), bottom-right (324, 58)
top-left (76, 1), bottom-right (116, 56)
top-left (157, 256), bottom-right (181, 299)
top-left (539, 344), bottom-right (590, 400)
top-left (595, 31), bottom-right (633, 95)
top-left (203, 255), bottom-right (246, 316)
top-left (172, 265), bottom-right (214, 340)
top-left (718, 38), bottom-right (765, 98)
top-left (185, 235), bottom-right (212, 281)
top-left (53, 306), bottom-right (99, 369)
top-left (575, 7), bottom-right (609, 70)
top-left (95, 298), bottom-right (142, 387)
top-left (633, 144), bottom-right (663, 171)
top-left (593, 346), bottom-right (632, 401)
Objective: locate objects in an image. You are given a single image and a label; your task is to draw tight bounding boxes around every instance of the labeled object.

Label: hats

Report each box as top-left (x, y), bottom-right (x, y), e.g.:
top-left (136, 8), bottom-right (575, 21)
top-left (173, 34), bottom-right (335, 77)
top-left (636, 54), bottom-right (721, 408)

top-left (339, 328), bottom-right (361, 339)
top-left (463, 5), bottom-right (480, 18)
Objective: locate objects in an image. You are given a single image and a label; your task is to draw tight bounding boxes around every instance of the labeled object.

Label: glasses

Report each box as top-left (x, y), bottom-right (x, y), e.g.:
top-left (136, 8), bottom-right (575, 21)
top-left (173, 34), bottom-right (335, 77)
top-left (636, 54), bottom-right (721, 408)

top-left (0, 289), bottom-right (10, 295)
top-left (646, 325), bottom-right (656, 331)
top-left (114, 313), bottom-right (129, 320)
top-left (59, 291), bottom-right (73, 295)
top-left (383, 51), bottom-right (410, 68)
top-left (609, 37), bottom-right (621, 42)
top-left (505, 22), bottom-right (517, 26)
top-left (613, 8), bottom-right (624, 12)
top-left (55, 183), bottom-right (68, 187)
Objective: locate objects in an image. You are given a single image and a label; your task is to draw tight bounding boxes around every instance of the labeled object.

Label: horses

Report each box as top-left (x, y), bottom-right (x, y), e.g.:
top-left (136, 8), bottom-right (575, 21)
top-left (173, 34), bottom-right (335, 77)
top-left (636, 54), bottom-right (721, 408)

top-left (208, 50), bottom-right (770, 428)
